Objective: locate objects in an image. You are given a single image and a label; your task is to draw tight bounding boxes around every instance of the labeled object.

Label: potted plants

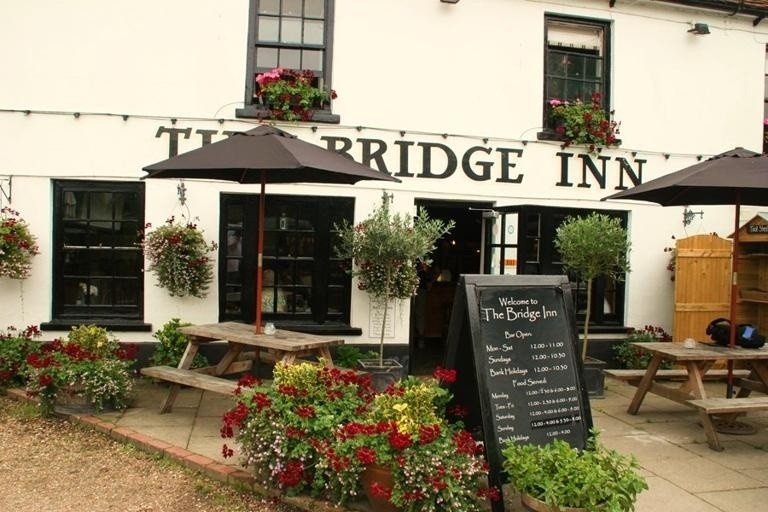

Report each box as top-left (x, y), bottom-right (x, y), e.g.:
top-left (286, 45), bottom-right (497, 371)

top-left (0, 204), bottom-right (40, 279)
top-left (325, 186), bottom-right (457, 399)
top-left (495, 428), bottom-right (651, 512)
top-left (551, 207), bottom-right (634, 400)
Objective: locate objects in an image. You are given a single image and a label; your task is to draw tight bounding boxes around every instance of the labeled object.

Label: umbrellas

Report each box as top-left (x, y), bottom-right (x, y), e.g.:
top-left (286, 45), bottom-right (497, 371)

top-left (138, 123), bottom-right (402, 379)
top-left (600, 146), bottom-right (768, 428)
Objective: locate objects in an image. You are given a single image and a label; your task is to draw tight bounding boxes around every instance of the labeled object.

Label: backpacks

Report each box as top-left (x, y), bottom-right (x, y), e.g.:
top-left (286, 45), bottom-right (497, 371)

top-left (705, 318), bottom-right (764, 348)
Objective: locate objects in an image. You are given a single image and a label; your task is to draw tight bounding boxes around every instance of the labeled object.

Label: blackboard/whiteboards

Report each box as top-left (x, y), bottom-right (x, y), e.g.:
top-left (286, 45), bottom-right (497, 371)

top-left (463, 274), bottom-right (592, 471)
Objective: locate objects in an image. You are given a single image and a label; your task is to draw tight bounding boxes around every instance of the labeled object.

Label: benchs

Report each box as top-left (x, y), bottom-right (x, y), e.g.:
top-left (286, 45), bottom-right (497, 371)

top-left (682, 393), bottom-right (768, 450)
top-left (600, 363), bottom-right (755, 381)
top-left (137, 363), bottom-right (278, 430)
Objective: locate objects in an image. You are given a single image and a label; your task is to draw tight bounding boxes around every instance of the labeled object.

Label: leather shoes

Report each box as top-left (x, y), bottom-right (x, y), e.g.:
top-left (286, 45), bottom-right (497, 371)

top-left (417, 336), bottom-right (424, 349)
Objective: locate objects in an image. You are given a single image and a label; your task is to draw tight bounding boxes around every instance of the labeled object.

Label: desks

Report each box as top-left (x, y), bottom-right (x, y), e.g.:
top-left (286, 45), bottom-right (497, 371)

top-left (626, 337), bottom-right (768, 452)
top-left (158, 319), bottom-right (345, 443)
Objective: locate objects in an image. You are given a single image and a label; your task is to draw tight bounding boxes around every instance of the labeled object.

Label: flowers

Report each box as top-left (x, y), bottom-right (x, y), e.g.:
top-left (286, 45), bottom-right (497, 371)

top-left (1, 320), bottom-right (144, 422)
top-left (546, 90), bottom-right (623, 154)
top-left (136, 191), bottom-right (219, 302)
top-left (611, 320), bottom-right (677, 370)
top-left (252, 61), bottom-right (337, 127)
top-left (219, 358), bottom-right (498, 512)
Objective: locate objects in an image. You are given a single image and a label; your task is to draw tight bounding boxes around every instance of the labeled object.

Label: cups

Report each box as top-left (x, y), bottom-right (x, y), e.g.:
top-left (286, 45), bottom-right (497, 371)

top-left (264, 322), bottom-right (276, 335)
